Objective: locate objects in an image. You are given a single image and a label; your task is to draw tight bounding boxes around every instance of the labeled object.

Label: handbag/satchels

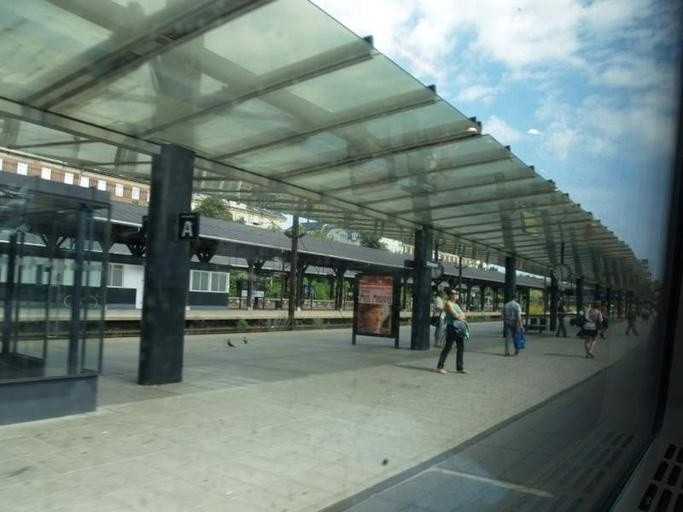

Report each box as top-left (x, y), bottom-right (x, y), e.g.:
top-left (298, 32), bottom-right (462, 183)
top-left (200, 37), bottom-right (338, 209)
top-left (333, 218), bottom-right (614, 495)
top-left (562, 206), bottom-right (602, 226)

top-left (430, 316), bottom-right (440, 327)
top-left (514, 326), bottom-right (526, 350)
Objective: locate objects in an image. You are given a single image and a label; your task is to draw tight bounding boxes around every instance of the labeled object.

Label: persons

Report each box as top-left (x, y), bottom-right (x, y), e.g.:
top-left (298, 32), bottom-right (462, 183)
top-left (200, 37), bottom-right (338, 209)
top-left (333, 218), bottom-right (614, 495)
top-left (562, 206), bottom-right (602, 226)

top-left (556, 299), bottom-right (657, 359)
top-left (437, 288), bottom-right (470, 374)
top-left (502, 295), bottom-right (522, 357)
top-left (432, 287), bottom-right (445, 349)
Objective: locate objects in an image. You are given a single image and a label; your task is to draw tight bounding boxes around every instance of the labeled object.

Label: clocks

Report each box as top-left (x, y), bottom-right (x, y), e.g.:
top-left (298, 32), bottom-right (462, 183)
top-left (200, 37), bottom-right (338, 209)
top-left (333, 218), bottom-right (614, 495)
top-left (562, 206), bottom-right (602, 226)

top-left (552, 263), bottom-right (571, 282)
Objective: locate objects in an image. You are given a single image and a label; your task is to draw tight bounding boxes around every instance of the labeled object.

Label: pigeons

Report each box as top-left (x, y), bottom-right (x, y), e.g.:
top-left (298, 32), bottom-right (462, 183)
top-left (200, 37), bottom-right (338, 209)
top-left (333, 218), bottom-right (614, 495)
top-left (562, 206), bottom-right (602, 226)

top-left (227, 338), bottom-right (237, 348)
top-left (243, 336), bottom-right (248, 344)
